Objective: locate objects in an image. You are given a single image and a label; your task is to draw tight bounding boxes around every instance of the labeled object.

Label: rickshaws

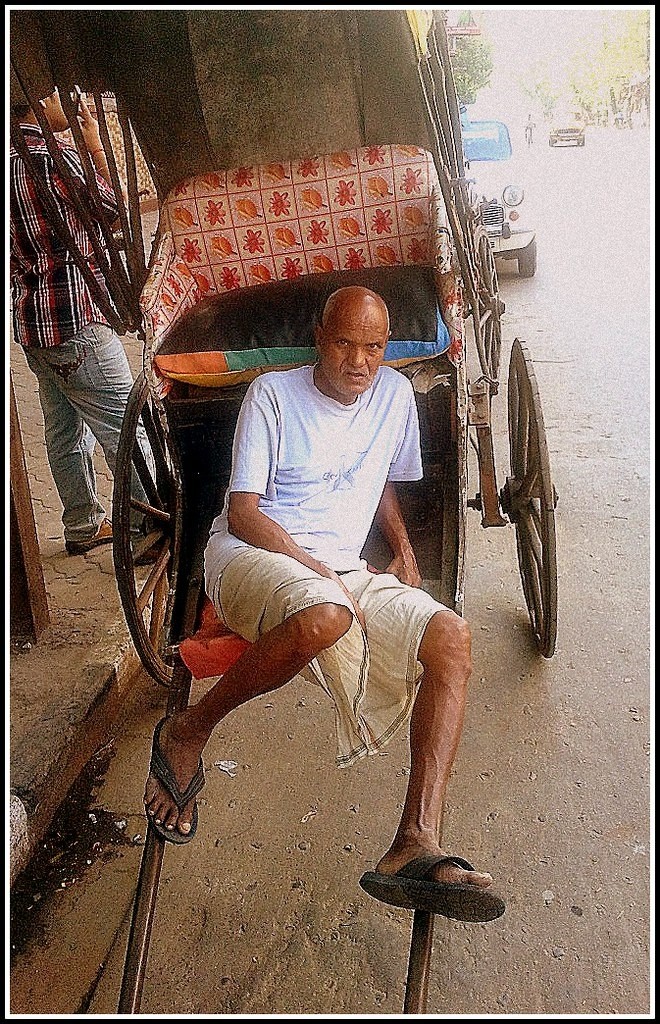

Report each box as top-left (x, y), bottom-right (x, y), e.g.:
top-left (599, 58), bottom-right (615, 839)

top-left (10, 11), bottom-right (560, 1015)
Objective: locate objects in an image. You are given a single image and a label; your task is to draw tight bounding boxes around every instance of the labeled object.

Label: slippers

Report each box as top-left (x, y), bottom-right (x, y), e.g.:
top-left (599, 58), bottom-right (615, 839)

top-left (359, 856), bottom-right (505, 922)
top-left (145, 716), bottom-right (206, 843)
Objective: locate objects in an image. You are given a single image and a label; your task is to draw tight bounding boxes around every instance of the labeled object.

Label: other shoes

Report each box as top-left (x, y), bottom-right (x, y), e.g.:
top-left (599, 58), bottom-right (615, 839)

top-left (65, 518), bottom-right (116, 555)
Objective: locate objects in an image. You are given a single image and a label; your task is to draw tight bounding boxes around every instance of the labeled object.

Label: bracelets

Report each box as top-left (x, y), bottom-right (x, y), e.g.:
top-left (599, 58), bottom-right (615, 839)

top-left (91, 148), bottom-right (105, 157)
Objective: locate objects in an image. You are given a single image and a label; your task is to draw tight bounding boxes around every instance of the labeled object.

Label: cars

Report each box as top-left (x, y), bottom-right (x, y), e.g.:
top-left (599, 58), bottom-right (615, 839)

top-left (547, 112), bottom-right (587, 148)
top-left (459, 121), bottom-right (539, 277)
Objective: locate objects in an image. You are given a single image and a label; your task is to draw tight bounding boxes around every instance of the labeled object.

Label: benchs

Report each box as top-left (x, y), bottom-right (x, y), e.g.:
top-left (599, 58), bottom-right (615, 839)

top-left (140, 142), bottom-right (453, 429)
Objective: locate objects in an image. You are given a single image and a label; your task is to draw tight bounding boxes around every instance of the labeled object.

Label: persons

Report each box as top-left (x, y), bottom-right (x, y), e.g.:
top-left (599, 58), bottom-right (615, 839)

top-left (9, 84), bottom-right (170, 562)
top-left (525, 114), bottom-right (535, 143)
top-left (146, 286), bottom-right (507, 924)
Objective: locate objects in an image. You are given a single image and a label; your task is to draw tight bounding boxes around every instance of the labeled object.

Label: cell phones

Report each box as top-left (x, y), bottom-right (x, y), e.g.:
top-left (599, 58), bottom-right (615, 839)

top-left (74, 84), bottom-right (81, 116)
